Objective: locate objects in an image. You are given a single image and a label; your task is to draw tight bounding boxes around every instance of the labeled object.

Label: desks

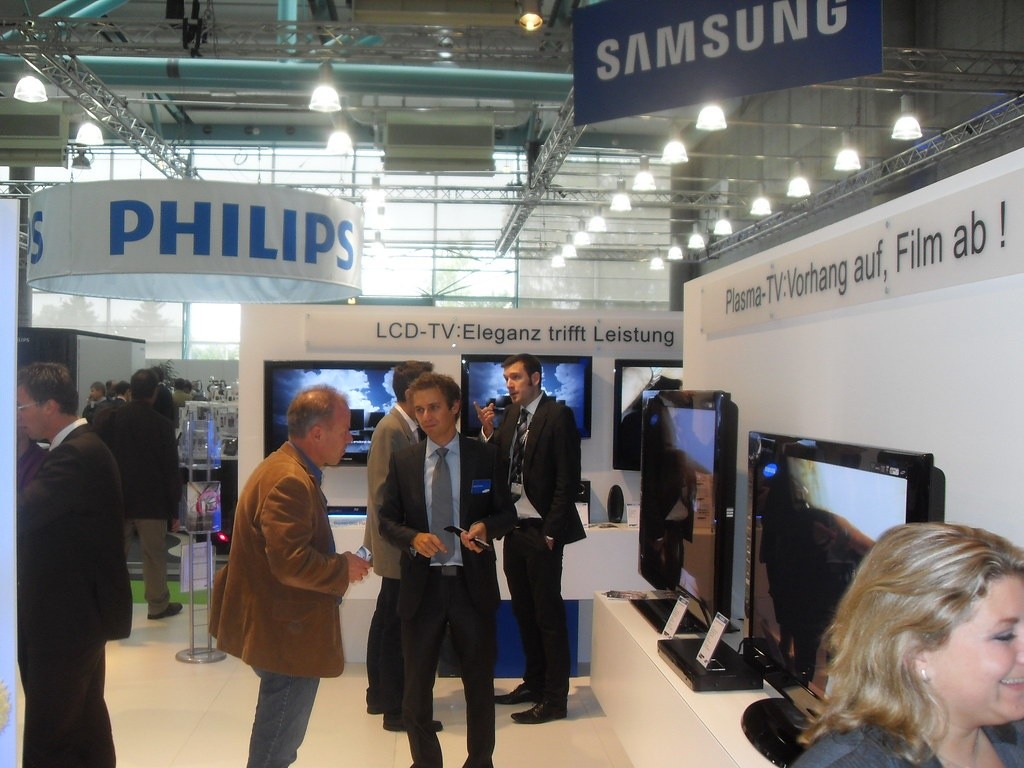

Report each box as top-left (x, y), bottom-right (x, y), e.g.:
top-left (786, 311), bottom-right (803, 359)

top-left (328, 521), bottom-right (655, 678)
top-left (590, 589), bottom-right (826, 768)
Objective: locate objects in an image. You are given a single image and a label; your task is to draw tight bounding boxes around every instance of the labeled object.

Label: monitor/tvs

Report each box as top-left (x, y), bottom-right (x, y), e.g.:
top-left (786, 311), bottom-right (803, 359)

top-left (635, 390), bottom-right (739, 633)
top-left (264, 360), bottom-right (430, 468)
top-left (741, 430), bottom-right (945, 768)
top-left (612, 359), bottom-right (683, 471)
top-left (460, 353), bottom-right (593, 438)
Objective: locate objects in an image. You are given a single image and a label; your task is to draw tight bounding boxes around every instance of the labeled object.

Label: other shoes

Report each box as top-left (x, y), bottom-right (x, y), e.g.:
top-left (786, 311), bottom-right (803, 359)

top-left (383, 719), bottom-right (443, 732)
top-left (147, 602), bottom-right (183, 619)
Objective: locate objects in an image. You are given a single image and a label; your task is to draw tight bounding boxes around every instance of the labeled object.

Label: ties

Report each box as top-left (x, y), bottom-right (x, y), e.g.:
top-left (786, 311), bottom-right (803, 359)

top-left (430, 447), bottom-right (454, 565)
top-left (510, 409), bottom-right (529, 503)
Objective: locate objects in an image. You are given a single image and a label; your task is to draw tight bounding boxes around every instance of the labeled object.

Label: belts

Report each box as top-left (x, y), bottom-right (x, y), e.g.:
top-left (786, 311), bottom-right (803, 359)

top-left (514, 518), bottom-right (542, 529)
top-left (438, 566), bottom-right (464, 577)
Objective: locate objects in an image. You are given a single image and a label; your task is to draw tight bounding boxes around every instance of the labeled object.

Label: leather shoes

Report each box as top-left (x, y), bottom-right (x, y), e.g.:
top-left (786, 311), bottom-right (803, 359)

top-left (511, 699), bottom-right (567, 724)
top-left (494, 683), bottom-right (547, 704)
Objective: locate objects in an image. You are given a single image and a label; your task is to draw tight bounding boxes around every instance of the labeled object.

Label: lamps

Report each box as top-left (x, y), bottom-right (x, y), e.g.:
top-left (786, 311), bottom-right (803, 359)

top-left (551, 95), bottom-right (923, 272)
top-left (71, 151), bottom-right (92, 170)
top-left (513, 0), bottom-right (544, 31)
top-left (244, 126), bottom-right (260, 136)
top-left (13, 52), bottom-right (49, 103)
top-left (307, 61), bottom-right (342, 113)
top-left (74, 103), bottom-right (105, 146)
top-left (324, 116), bottom-right (353, 155)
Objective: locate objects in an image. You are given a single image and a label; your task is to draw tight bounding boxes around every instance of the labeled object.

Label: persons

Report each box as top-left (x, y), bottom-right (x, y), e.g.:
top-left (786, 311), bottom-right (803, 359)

top-left (791, 522), bottom-right (1024, 768)
top-left (474, 354), bottom-right (587, 723)
top-left (362, 361), bottom-right (518, 768)
top-left (17, 362), bottom-right (133, 768)
top-left (80, 367), bottom-right (208, 621)
top-left (210, 386), bottom-right (371, 768)
